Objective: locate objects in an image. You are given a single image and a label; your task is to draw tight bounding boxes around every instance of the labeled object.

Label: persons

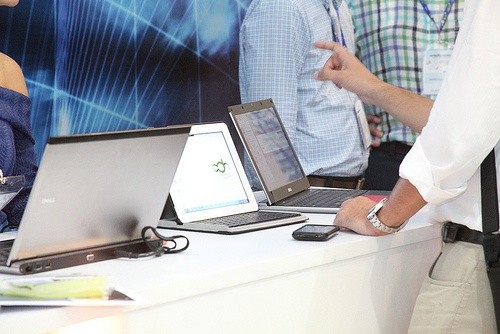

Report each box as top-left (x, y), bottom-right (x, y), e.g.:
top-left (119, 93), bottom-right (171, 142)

top-left (238, 0), bottom-right (371, 190)
top-left (313, 0), bottom-right (500, 333)
top-left (341, 1), bottom-right (463, 191)
top-left (0, 0), bottom-right (36, 233)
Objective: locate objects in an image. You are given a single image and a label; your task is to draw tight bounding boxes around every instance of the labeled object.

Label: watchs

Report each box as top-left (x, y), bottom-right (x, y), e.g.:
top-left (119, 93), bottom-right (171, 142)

top-left (367, 197), bottom-right (409, 233)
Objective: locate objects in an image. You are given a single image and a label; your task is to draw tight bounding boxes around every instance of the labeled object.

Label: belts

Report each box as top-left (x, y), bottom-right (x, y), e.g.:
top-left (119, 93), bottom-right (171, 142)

top-left (371, 141), bottom-right (412, 154)
top-left (441, 221), bottom-right (484, 244)
top-left (308, 176), bottom-right (366, 191)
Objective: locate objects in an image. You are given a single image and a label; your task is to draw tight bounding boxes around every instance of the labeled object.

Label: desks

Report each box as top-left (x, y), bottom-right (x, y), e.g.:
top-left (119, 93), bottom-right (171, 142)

top-left (0, 187), bottom-right (442, 334)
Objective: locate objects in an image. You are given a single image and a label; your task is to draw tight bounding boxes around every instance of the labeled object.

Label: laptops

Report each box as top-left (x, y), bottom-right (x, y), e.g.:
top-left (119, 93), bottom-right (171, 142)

top-left (227, 98), bottom-right (394, 213)
top-left (168, 121), bottom-right (309, 234)
top-left (0, 125), bottom-right (191, 275)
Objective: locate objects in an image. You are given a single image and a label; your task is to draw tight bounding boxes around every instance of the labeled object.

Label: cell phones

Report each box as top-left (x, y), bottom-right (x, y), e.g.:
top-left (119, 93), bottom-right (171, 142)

top-left (292, 224), bottom-right (341, 242)
